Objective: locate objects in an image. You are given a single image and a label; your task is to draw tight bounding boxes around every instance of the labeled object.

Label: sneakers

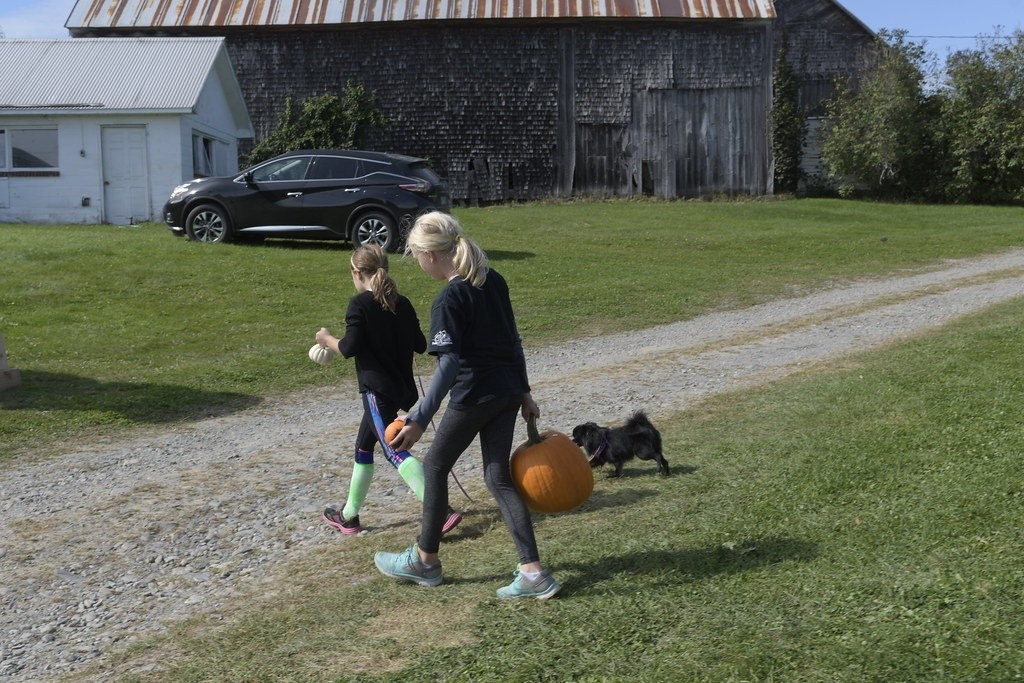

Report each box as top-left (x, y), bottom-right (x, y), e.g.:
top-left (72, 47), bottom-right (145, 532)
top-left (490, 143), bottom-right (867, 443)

top-left (443, 504), bottom-right (462, 536)
top-left (322, 503), bottom-right (360, 535)
top-left (374, 542), bottom-right (442, 587)
top-left (497, 563), bottom-right (561, 600)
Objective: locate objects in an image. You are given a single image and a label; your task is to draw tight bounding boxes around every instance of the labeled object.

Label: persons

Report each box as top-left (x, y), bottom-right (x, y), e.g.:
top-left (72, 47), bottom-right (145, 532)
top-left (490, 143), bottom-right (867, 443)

top-left (315, 245), bottom-right (462, 536)
top-left (374, 210), bottom-right (561, 600)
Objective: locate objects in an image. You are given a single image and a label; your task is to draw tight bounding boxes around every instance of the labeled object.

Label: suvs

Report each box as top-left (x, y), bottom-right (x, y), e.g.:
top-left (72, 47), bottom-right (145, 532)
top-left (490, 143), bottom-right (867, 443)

top-left (161, 148), bottom-right (452, 255)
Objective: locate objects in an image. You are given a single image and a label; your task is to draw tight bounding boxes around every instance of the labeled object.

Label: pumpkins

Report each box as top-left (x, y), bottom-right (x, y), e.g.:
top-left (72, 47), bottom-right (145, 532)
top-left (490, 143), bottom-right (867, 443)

top-left (384, 420), bottom-right (405, 449)
top-left (510, 414), bottom-right (594, 515)
top-left (309, 344), bottom-right (334, 365)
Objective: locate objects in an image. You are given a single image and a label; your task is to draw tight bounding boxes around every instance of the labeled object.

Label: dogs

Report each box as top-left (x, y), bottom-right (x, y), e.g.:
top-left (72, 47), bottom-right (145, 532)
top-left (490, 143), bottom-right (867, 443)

top-left (571, 409), bottom-right (671, 480)
